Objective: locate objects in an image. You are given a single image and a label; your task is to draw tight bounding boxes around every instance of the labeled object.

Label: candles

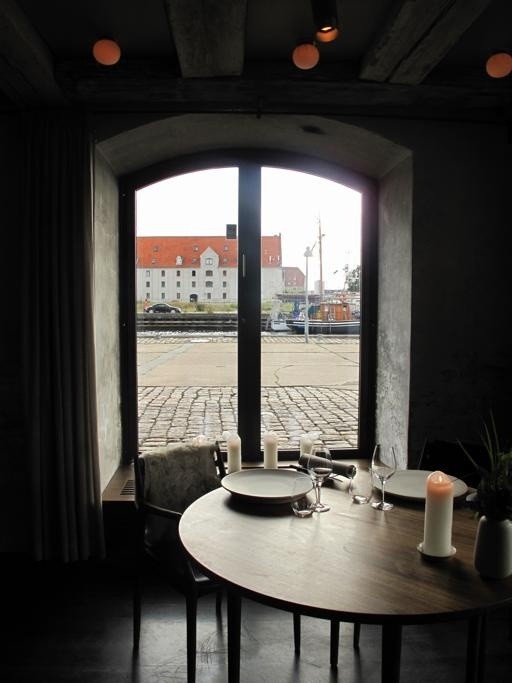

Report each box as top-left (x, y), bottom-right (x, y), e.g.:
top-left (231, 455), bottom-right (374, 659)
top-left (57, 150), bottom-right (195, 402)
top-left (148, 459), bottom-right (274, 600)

top-left (226, 434), bottom-right (242, 473)
top-left (263, 430), bottom-right (279, 469)
top-left (299, 434), bottom-right (315, 457)
top-left (422, 469), bottom-right (455, 555)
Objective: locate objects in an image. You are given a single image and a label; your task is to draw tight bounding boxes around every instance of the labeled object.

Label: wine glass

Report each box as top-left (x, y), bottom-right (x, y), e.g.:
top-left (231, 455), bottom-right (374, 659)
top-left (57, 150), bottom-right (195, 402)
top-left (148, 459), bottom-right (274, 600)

top-left (307, 446), bottom-right (334, 512)
top-left (372, 443), bottom-right (397, 511)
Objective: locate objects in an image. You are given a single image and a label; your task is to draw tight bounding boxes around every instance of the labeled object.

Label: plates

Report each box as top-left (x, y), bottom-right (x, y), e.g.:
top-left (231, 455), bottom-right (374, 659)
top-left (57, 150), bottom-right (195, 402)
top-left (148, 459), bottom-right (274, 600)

top-left (370, 468), bottom-right (469, 500)
top-left (221, 468), bottom-right (313, 505)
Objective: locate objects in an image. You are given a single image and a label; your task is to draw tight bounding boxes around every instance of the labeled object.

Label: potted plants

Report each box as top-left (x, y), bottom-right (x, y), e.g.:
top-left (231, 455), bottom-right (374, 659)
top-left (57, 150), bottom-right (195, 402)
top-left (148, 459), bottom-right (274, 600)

top-left (453, 407), bottom-right (512, 581)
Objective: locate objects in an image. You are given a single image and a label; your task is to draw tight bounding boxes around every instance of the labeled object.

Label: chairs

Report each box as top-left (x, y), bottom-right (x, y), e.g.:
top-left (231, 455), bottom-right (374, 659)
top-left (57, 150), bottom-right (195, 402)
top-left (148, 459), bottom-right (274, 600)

top-left (133, 437), bottom-right (228, 682)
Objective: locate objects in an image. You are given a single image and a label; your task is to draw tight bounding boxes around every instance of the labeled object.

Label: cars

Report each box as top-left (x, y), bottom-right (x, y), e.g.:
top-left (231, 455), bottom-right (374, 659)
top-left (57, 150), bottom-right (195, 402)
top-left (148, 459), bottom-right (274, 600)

top-left (144, 303), bottom-right (182, 314)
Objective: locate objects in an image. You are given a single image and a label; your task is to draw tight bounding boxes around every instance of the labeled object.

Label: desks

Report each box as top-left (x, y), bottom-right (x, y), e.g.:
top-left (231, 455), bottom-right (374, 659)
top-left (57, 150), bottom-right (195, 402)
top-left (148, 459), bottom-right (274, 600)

top-left (178, 464), bottom-right (512, 682)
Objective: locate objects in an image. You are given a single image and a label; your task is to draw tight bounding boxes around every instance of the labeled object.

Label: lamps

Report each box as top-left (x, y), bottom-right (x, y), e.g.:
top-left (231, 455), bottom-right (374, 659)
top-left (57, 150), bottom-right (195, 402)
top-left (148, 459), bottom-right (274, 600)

top-left (484, 46), bottom-right (511, 80)
top-left (91, 31), bottom-right (122, 66)
top-left (290, 0), bottom-right (341, 72)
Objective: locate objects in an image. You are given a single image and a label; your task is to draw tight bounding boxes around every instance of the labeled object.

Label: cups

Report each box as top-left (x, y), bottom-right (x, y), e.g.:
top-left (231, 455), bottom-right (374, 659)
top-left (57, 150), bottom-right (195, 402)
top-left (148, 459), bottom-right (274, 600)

top-left (290, 476), bottom-right (316, 517)
top-left (349, 466), bottom-right (373, 504)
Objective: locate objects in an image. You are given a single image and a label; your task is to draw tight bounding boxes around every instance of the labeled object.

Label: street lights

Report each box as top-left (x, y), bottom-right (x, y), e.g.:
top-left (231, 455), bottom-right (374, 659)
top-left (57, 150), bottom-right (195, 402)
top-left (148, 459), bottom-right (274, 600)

top-left (302, 247), bottom-right (313, 342)
